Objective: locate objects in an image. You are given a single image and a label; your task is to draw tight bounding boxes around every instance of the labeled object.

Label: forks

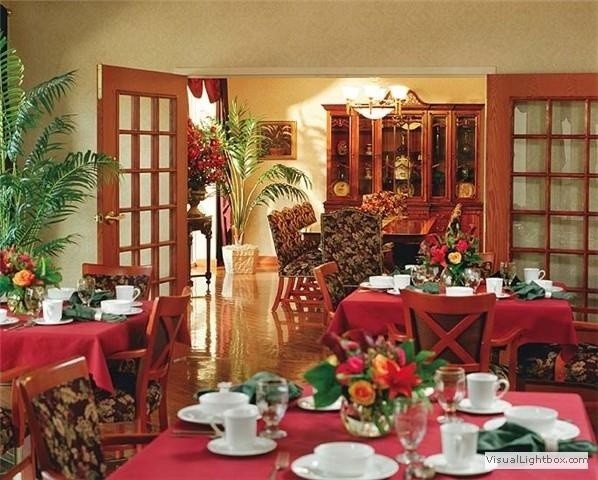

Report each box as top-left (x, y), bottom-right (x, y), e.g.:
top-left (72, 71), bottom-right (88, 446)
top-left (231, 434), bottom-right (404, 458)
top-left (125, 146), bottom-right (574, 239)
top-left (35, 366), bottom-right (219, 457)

top-left (267, 451), bottom-right (290, 480)
top-left (3, 321), bottom-right (25, 334)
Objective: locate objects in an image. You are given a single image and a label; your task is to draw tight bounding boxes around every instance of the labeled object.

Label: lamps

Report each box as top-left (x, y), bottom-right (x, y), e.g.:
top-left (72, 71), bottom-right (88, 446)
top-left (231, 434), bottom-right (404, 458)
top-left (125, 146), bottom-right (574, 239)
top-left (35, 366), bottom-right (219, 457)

top-left (342, 85), bottom-right (410, 119)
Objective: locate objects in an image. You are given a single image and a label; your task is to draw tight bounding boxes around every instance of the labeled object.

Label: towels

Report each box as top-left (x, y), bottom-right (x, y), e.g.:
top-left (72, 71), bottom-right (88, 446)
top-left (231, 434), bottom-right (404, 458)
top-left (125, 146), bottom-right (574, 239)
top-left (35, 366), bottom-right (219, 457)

top-left (367, 268), bottom-right (415, 287)
top-left (421, 282), bottom-right (443, 295)
top-left (70, 290), bottom-right (112, 306)
top-left (195, 372), bottom-right (301, 408)
top-left (515, 282), bottom-right (574, 302)
top-left (493, 272), bottom-right (521, 287)
top-left (62, 305), bottom-right (126, 324)
top-left (477, 423), bottom-right (597, 459)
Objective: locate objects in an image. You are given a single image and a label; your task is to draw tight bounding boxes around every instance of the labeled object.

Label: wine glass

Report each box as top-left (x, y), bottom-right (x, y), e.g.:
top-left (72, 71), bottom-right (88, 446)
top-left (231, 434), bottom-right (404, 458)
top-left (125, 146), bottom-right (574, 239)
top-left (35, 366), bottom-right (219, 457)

top-left (393, 398), bottom-right (427, 465)
top-left (499, 261), bottom-right (517, 292)
top-left (255, 379), bottom-right (289, 439)
top-left (77, 278), bottom-right (95, 322)
top-left (25, 286), bottom-right (44, 319)
top-left (433, 366), bottom-right (464, 425)
top-left (8, 289), bottom-right (24, 317)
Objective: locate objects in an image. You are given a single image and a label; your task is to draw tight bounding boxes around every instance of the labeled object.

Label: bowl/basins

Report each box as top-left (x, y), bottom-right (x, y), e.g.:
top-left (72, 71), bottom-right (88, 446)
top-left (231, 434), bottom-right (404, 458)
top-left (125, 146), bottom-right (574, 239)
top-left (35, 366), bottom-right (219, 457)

top-left (368, 275), bottom-right (393, 286)
top-left (198, 391), bottom-right (249, 419)
top-left (503, 405), bottom-right (559, 440)
top-left (315, 442), bottom-right (375, 477)
top-left (446, 287), bottom-right (473, 296)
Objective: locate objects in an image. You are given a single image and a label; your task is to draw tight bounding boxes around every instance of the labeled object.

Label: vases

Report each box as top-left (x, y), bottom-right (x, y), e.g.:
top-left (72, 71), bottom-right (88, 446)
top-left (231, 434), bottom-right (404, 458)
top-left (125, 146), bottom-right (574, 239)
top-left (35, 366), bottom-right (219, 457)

top-left (440, 265), bottom-right (480, 292)
top-left (188, 188), bottom-right (208, 217)
top-left (7, 288), bottom-right (44, 316)
top-left (341, 397), bottom-right (396, 437)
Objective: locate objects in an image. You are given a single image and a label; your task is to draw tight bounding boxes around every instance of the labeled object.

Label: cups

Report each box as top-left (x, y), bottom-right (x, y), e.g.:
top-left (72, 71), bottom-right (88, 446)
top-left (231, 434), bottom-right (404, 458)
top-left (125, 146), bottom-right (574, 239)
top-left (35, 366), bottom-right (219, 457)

top-left (0, 310), bottom-right (7, 322)
top-left (466, 372), bottom-right (510, 411)
top-left (116, 286), bottom-right (141, 303)
top-left (410, 264), bottom-right (427, 288)
top-left (110, 299), bottom-right (131, 313)
top-left (425, 263), bottom-right (439, 283)
top-left (101, 300), bottom-right (114, 314)
top-left (60, 287), bottom-right (74, 300)
top-left (485, 277), bottom-right (503, 295)
top-left (439, 422), bottom-right (479, 466)
top-left (47, 288), bottom-right (59, 298)
top-left (393, 274), bottom-right (410, 288)
top-left (523, 267), bottom-right (545, 284)
top-left (538, 279), bottom-right (553, 298)
top-left (405, 265), bottom-right (417, 271)
top-left (40, 299), bottom-right (63, 323)
top-left (210, 408), bottom-right (260, 450)
top-left (463, 267), bottom-right (481, 295)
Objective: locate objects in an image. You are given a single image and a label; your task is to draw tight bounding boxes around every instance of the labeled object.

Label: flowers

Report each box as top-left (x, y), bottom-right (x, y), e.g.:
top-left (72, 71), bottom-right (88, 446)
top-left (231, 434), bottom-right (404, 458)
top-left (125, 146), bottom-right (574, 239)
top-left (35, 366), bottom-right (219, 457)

top-left (0, 243), bottom-right (63, 313)
top-left (187, 118), bottom-right (228, 190)
top-left (304, 330), bottom-right (450, 435)
top-left (419, 218), bottom-right (484, 286)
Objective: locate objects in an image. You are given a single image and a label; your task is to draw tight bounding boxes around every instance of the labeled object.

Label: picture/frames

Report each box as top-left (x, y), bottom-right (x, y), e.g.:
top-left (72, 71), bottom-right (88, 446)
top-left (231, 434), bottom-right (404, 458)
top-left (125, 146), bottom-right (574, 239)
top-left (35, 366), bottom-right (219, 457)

top-left (256, 120), bottom-right (297, 160)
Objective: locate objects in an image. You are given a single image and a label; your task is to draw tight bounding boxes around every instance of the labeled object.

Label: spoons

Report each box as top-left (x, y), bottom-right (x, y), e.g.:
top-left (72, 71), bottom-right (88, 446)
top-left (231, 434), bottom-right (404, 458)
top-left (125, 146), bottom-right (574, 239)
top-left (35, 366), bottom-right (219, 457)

top-left (10, 321), bottom-right (36, 333)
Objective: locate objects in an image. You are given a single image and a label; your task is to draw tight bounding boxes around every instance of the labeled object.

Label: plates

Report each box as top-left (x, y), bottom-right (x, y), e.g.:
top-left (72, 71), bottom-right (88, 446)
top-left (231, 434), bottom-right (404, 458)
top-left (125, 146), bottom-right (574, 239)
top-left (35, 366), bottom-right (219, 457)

top-left (207, 436), bottom-right (277, 457)
top-left (290, 452), bottom-right (397, 479)
top-left (552, 286), bottom-right (563, 292)
top-left (177, 403), bottom-right (262, 425)
top-left (35, 317), bottom-right (73, 325)
top-left (110, 307), bottom-right (142, 316)
top-left (455, 398), bottom-right (513, 415)
top-left (0, 316), bottom-right (19, 326)
top-left (131, 300), bottom-right (143, 307)
top-left (495, 292), bottom-right (511, 298)
top-left (482, 416), bottom-right (581, 443)
top-left (359, 281), bottom-right (394, 292)
top-left (297, 393), bottom-right (347, 411)
top-left (422, 452), bottom-right (497, 476)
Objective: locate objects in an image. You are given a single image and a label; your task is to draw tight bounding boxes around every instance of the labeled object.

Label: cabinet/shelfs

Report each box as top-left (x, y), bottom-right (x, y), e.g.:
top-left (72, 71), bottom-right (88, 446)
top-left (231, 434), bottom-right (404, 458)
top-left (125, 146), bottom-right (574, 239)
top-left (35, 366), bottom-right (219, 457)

top-left (326, 206), bottom-right (361, 212)
top-left (376, 110), bottom-right (427, 202)
top-left (428, 109), bottom-right (482, 203)
top-left (331, 110), bottom-right (374, 200)
top-left (438, 211), bottom-right (481, 252)
top-left (407, 209), bottom-right (429, 218)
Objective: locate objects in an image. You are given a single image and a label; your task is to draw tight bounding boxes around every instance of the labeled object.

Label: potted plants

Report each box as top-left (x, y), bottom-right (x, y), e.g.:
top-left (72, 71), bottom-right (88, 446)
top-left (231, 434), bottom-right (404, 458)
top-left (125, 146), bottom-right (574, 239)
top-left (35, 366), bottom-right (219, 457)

top-left (200, 95), bottom-right (311, 273)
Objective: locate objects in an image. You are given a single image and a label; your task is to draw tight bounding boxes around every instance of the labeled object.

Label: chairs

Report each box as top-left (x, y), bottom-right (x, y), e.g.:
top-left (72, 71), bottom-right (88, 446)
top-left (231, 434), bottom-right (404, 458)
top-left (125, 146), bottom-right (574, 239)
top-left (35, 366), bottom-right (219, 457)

top-left (82, 263), bottom-right (153, 434)
top-left (313, 261), bottom-right (346, 326)
top-left (96, 295), bottom-right (190, 459)
top-left (267, 202), bottom-right (322, 312)
top-left (0, 357), bottom-right (160, 480)
top-left (321, 209), bottom-right (383, 294)
top-left (517, 306), bottom-right (598, 391)
top-left (384, 288), bottom-right (522, 391)
